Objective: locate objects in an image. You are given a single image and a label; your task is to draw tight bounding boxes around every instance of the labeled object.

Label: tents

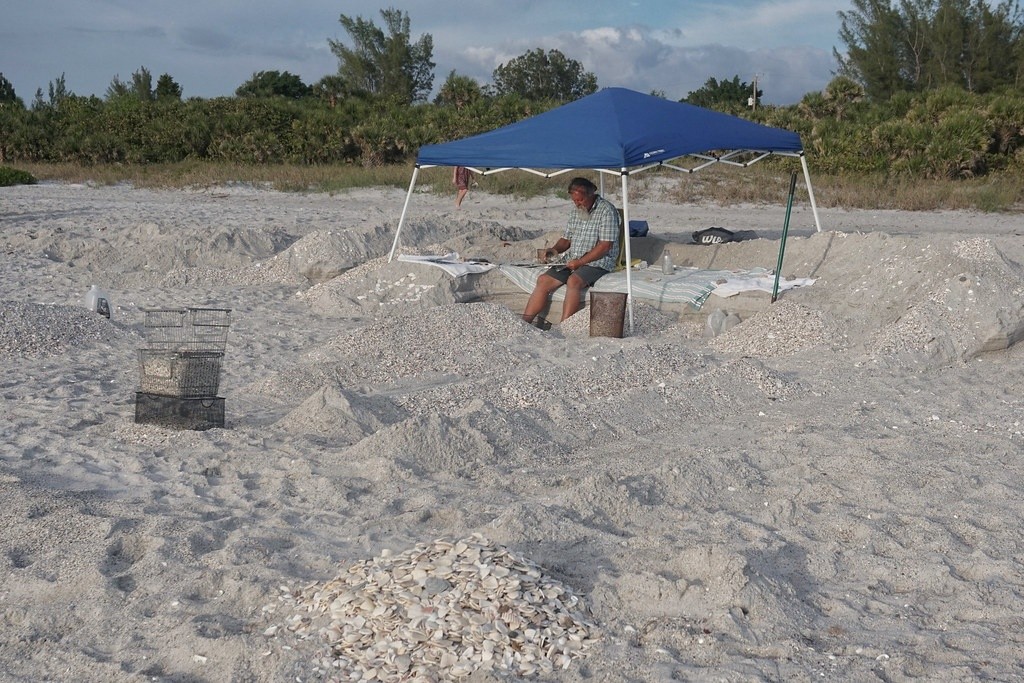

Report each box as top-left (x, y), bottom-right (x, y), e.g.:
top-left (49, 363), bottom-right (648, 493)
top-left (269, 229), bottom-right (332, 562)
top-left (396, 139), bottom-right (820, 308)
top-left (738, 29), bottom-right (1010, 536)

top-left (388, 87), bottom-right (822, 333)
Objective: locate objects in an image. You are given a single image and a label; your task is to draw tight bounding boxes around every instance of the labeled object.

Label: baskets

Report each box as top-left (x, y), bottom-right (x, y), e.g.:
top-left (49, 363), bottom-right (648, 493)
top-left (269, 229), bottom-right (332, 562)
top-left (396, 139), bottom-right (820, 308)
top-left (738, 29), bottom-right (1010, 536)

top-left (142, 308), bottom-right (231, 353)
top-left (137, 346), bottom-right (225, 399)
top-left (517, 314), bottom-right (552, 331)
top-left (134, 391), bottom-right (226, 431)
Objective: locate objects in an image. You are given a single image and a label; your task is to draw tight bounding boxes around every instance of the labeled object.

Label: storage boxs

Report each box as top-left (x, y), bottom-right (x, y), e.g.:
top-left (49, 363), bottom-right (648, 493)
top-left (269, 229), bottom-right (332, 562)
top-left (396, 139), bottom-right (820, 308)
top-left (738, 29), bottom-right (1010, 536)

top-left (133, 391), bottom-right (225, 430)
top-left (137, 306), bottom-right (230, 352)
top-left (135, 346), bottom-right (223, 396)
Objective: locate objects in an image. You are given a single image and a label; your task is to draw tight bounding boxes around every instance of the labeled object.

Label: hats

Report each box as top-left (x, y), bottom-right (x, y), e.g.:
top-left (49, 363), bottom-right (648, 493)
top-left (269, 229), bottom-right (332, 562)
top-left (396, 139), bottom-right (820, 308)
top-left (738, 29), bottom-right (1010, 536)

top-left (573, 177), bottom-right (597, 191)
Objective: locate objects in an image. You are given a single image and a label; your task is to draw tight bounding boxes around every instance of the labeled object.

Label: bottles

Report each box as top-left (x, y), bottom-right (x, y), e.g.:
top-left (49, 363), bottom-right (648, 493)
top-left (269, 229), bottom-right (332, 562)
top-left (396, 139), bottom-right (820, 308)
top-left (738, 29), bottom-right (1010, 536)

top-left (662, 250), bottom-right (674, 275)
top-left (85, 285), bottom-right (113, 321)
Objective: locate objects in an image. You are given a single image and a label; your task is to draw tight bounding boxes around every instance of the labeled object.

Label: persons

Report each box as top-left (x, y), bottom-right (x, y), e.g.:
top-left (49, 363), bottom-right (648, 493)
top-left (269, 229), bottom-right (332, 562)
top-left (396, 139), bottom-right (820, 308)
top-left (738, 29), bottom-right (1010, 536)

top-left (452, 166), bottom-right (478, 210)
top-left (522, 178), bottom-right (622, 323)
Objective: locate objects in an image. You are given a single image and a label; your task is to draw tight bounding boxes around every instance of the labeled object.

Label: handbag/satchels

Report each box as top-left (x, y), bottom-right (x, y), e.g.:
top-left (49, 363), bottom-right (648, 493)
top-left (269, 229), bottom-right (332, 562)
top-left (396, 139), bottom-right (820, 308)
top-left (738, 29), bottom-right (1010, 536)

top-left (733, 230), bottom-right (759, 242)
top-left (628, 220), bottom-right (649, 237)
top-left (471, 181), bottom-right (479, 189)
top-left (691, 227), bottom-right (734, 245)
